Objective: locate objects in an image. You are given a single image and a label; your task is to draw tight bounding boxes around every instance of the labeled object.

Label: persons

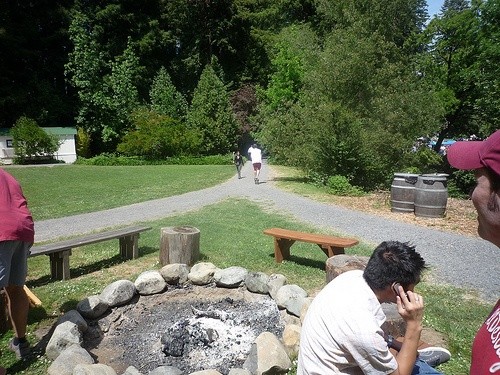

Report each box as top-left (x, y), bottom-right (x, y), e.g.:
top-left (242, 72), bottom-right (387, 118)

top-left (0, 168), bottom-right (34, 360)
top-left (248, 144), bottom-right (262, 184)
top-left (296, 240), bottom-right (451, 375)
top-left (447, 129), bottom-right (500, 375)
top-left (233, 151), bottom-right (244, 179)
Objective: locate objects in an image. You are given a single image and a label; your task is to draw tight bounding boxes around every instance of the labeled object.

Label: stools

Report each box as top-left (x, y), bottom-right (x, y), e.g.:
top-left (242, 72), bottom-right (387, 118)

top-left (324, 255), bottom-right (371, 284)
top-left (377, 302), bottom-right (406, 338)
top-left (159, 224), bottom-right (201, 267)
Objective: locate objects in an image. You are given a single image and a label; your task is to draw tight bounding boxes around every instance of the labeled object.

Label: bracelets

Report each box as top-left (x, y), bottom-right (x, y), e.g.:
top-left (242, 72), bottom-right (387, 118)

top-left (387, 335), bottom-right (394, 347)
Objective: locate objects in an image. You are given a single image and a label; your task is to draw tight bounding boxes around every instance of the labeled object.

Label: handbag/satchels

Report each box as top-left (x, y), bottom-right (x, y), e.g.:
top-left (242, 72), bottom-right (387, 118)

top-left (235, 161), bottom-right (240, 165)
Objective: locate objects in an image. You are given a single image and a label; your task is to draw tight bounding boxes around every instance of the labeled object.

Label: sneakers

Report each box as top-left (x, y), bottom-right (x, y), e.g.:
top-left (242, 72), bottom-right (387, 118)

top-left (8, 338), bottom-right (31, 360)
top-left (417, 347), bottom-right (452, 369)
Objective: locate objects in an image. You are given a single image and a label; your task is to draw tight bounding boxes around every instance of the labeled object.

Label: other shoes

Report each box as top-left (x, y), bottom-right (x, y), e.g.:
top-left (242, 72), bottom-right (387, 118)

top-left (254, 178), bottom-right (259, 184)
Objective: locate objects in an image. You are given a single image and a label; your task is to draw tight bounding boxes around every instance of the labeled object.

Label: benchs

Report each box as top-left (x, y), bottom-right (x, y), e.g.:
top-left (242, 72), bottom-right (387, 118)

top-left (263, 227), bottom-right (360, 264)
top-left (29, 224), bottom-right (153, 280)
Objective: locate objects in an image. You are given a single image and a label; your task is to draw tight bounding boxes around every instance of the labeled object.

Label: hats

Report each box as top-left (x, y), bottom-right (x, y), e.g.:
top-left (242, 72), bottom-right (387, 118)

top-left (446, 130), bottom-right (500, 175)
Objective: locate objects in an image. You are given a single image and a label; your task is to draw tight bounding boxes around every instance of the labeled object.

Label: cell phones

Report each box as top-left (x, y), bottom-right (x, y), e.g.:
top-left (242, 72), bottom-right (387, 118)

top-left (391, 281), bottom-right (411, 305)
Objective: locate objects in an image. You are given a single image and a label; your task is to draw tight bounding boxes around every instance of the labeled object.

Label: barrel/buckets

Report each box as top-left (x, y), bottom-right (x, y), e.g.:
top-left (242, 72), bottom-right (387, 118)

top-left (415, 174), bottom-right (447, 215)
top-left (391, 173), bottom-right (419, 213)
